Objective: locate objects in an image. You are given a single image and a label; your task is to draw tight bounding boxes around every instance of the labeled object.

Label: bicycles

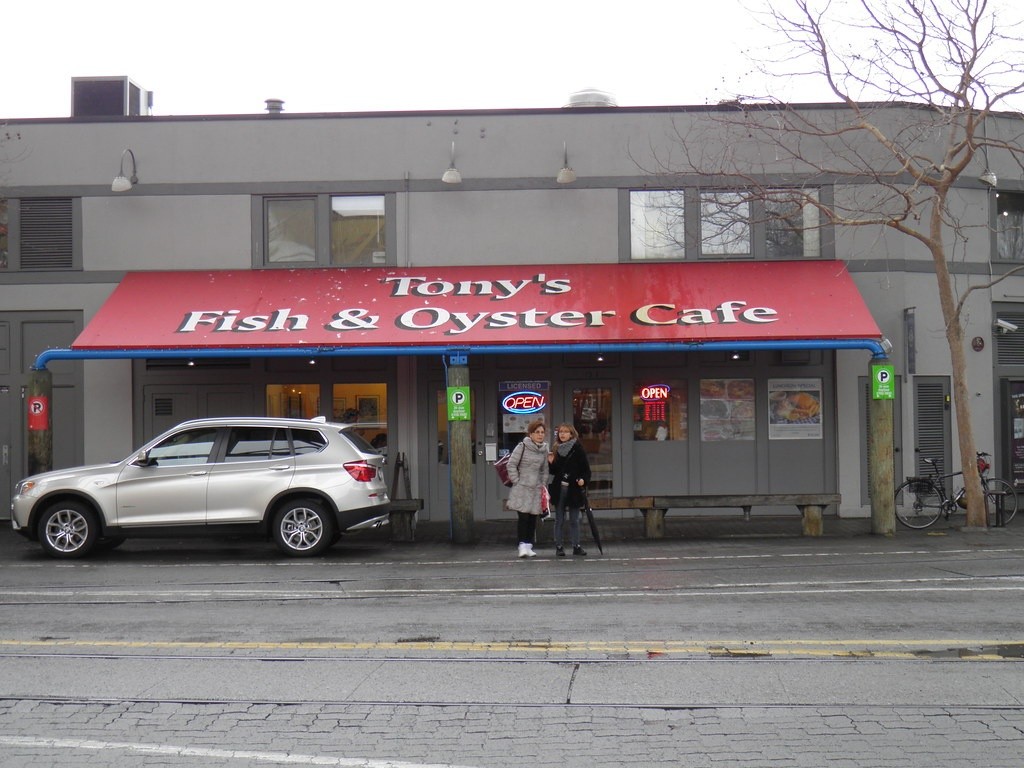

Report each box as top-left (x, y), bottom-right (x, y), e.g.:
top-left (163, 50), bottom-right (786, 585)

top-left (894, 451), bottom-right (1018, 529)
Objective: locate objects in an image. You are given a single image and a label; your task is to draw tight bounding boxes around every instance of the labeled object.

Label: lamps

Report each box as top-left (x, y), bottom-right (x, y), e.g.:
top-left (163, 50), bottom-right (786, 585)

top-left (111, 149), bottom-right (139, 193)
top-left (557, 140), bottom-right (576, 184)
top-left (442, 141), bottom-right (463, 185)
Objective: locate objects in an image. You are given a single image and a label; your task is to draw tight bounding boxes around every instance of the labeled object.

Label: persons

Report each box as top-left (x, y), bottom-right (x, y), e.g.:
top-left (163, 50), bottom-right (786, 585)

top-left (505, 420), bottom-right (552, 559)
top-left (338, 409), bottom-right (360, 423)
top-left (371, 433), bottom-right (387, 451)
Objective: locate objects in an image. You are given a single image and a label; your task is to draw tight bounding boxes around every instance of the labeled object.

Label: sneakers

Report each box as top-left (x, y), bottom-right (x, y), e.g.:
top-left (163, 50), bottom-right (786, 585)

top-left (518, 542), bottom-right (536, 557)
top-left (573, 544), bottom-right (587, 555)
top-left (556, 546), bottom-right (565, 556)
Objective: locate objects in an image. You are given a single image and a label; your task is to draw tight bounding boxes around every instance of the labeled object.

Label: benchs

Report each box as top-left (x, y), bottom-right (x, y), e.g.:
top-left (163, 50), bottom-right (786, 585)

top-left (584, 492), bottom-right (843, 537)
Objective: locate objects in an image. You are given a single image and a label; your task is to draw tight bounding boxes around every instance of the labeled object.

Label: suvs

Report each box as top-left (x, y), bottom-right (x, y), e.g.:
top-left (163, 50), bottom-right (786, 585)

top-left (7, 416), bottom-right (393, 557)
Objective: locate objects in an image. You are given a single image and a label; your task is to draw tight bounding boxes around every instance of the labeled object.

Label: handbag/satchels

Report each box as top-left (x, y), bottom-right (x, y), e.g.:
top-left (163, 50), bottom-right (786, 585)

top-left (494, 453), bottom-right (520, 488)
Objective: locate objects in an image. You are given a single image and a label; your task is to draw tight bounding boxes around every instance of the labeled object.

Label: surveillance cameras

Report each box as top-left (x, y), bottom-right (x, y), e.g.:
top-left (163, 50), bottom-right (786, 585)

top-left (993, 319), bottom-right (1019, 334)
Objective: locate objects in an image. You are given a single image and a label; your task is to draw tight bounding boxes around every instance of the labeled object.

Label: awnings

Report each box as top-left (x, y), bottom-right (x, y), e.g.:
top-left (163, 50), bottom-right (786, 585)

top-left (546, 422), bottom-right (592, 556)
top-left (68, 260), bottom-right (885, 346)
